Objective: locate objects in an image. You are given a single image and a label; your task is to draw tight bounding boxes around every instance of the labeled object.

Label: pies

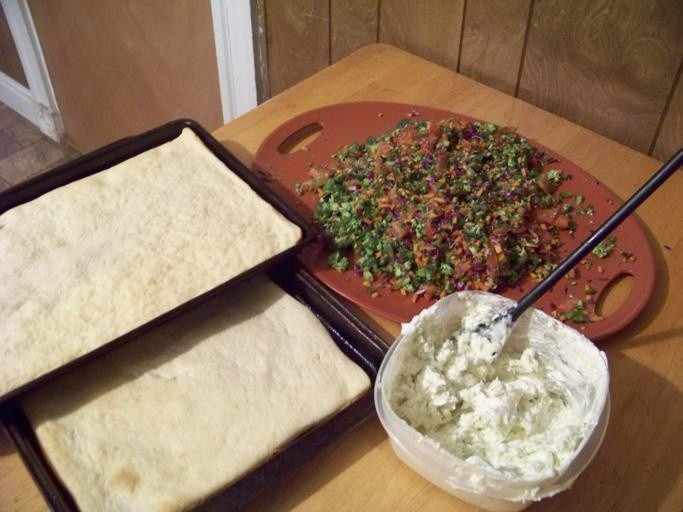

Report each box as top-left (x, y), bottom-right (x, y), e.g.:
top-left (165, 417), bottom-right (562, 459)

top-left (0, 127), bottom-right (303, 395)
top-left (15, 272), bottom-right (371, 512)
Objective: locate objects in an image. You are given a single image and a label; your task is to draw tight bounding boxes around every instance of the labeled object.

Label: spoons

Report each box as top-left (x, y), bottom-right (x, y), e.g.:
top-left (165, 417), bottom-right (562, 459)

top-left (445, 146), bottom-right (683, 366)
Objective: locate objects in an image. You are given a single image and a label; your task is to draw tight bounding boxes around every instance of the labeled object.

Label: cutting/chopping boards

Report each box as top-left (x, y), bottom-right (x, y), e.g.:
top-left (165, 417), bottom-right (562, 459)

top-left (252, 101), bottom-right (656, 343)
